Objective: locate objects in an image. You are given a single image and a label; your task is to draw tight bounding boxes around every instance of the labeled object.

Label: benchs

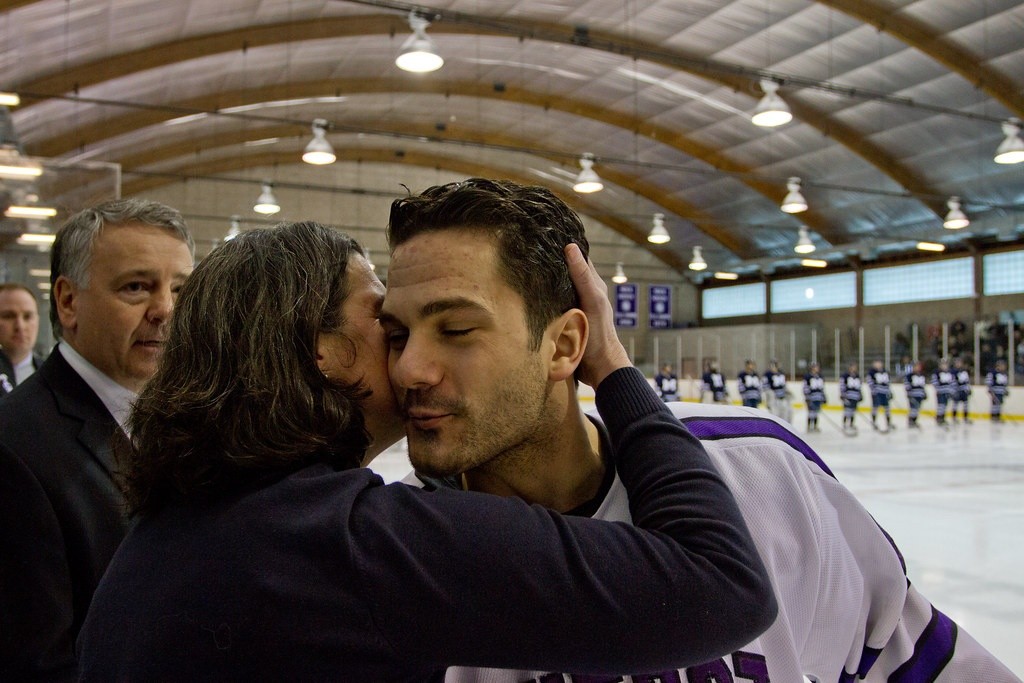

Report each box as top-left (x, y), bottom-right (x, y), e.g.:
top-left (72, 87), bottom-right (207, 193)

top-left (825, 342), bottom-right (940, 378)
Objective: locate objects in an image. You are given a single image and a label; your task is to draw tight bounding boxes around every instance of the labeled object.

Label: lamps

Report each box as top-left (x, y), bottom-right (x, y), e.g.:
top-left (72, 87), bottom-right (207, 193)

top-left (225, 215), bottom-right (242, 241)
top-left (0, 91), bottom-right (21, 107)
top-left (794, 227), bottom-right (817, 254)
top-left (395, 13), bottom-right (443, 74)
top-left (993, 119), bottom-right (1024, 165)
top-left (688, 246), bottom-right (707, 270)
top-left (3, 204), bottom-right (59, 221)
top-left (252, 181), bottom-right (280, 213)
top-left (573, 155), bottom-right (603, 193)
top-left (751, 77), bottom-right (794, 126)
top-left (647, 214), bottom-right (671, 244)
top-left (780, 178), bottom-right (809, 213)
top-left (0, 153), bottom-right (42, 182)
top-left (16, 232), bottom-right (57, 247)
top-left (302, 119), bottom-right (336, 166)
top-left (943, 196), bottom-right (969, 229)
top-left (611, 262), bottom-right (628, 284)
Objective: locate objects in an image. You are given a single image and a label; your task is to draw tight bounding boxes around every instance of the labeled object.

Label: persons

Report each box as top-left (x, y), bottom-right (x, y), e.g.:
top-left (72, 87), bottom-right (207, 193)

top-left (905, 362), bottom-right (927, 427)
top-left (738, 358), bottom-right (793, 420)
top-left (701, 361), bottom-right (729, 405)
top-left (804, 363), bottom-right (826, 433)
top-left (986, 360), bottom-right (1009, 423)
top-left (0, 283), bottom-right (43, 397)
top-left (654, 364), bottom-right (679, 403)
top-left (896, 354), bottom-right (910, 382)
top-left (69, 220), bottom-right (779, 683)
top-left (0, 200), bottom-right (196, 683)
top-left (932, 359), bottom-right (973, 424)
top-left (868, 359), bottom-right (896, 429)
top-left (840, 362), bottom-right (863, 430)
top-left (381, 177), bottom-right (1024, 683)
